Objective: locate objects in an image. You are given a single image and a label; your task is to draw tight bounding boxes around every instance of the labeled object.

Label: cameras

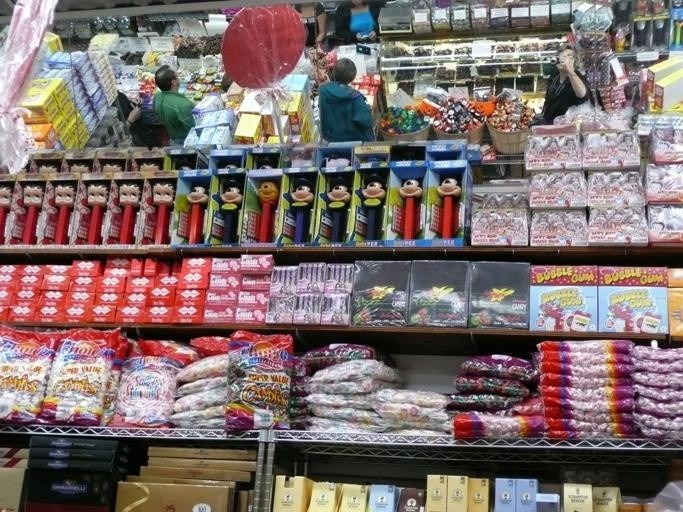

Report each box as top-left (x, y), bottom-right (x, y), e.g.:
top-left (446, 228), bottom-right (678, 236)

top-left (550, 56), bottom-right (560, 65)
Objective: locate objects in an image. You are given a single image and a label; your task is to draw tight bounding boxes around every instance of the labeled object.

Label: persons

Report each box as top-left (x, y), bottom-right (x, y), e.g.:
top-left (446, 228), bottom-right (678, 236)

top-left (293, 2), bottom-right (327, 49)
top-left (334, 0), bottom-right (381, 44)
top-left (538, 41), bottom-right (590, 125)
top-left (151, 64), bottom-right (195, 145)
top-left (117, 90), bottom-right (147, 146)
top-left (316, 57), bottom-right (374, 142)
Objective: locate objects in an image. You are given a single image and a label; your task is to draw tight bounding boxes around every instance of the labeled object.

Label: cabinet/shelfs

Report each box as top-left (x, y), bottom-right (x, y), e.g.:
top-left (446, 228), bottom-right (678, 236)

top-left (1, 120), bottom-right (681, 511)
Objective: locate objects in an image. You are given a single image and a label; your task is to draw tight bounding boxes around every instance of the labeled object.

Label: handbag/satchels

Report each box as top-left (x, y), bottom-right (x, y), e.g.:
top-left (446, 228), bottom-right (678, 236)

top-left (327, 36), bottom-right (349, 50)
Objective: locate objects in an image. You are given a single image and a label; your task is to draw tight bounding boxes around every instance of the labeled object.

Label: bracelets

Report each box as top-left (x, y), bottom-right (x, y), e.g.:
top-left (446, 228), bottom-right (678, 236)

top-left (126, 119), bottom-right (133, 125)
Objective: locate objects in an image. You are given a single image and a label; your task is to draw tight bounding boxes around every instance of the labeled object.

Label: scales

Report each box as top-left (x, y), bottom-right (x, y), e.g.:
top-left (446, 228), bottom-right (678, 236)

top-left (378, 7), bottom-right (413, 34)
